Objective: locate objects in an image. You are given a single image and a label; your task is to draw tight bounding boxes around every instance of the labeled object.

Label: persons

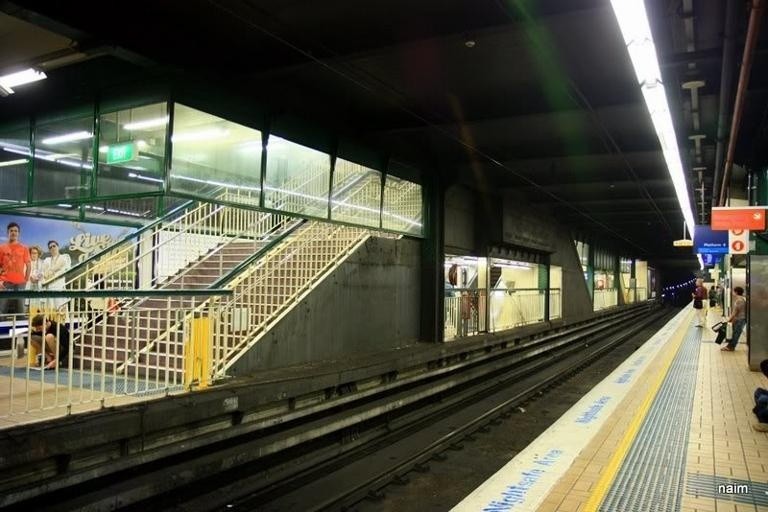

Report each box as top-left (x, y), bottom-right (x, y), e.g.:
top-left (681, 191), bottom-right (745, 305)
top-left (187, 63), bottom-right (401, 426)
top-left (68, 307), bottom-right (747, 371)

top-left (31, 313), bottom-right (74, 371)
top-left (42, 240), bottom-right (72, 312)
top-left (709, 286), bottom-right (716, 306)
top-left (721, 285), bottom-right (747, 351)
top-left (25, 244), bottom-right (44, 316)
top-left (0, 222), bottom-right (31, 321)
top-left (691, 278), bottom-right (708, 328)
top-left (455, 290), bottom-right (471, 336)
top-left (86, 273), bottom-right (110, 329)
top-left (752, 356), bottom-right (768, 432)
top-left (720, 285), bottom-right (724, 317)
top-left (716, 285), bottom-right (721, 306)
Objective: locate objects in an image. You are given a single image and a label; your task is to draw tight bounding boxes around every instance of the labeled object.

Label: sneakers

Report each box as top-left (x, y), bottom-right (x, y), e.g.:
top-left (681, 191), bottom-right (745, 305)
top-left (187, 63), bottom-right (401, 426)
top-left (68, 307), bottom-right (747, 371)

top-left (752, 423), bottom-right (768, 431)
top-left (722, 345), bottom-right (735, 351)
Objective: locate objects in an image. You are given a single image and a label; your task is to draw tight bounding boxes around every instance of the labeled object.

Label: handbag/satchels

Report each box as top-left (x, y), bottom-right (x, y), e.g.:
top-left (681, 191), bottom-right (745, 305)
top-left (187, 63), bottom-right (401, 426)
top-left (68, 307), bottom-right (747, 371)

top-left (693, 300), bottom-right (702, 309)
top-left (715, 322), bottom-right (732, 344)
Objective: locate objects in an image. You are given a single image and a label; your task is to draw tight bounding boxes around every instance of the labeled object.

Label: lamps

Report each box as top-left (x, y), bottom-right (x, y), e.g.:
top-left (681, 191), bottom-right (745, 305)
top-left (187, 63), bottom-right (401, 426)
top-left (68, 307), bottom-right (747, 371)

top-left (1, 63), bottom-right (48, 94)
top-left (605, 0), bottom-right (707, 273)
